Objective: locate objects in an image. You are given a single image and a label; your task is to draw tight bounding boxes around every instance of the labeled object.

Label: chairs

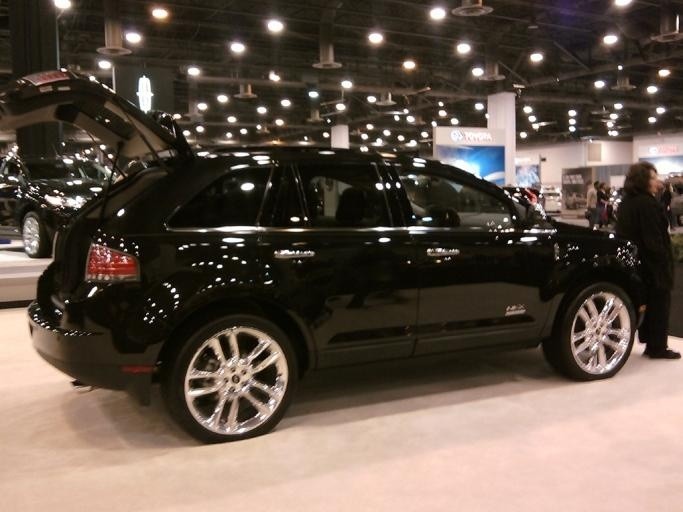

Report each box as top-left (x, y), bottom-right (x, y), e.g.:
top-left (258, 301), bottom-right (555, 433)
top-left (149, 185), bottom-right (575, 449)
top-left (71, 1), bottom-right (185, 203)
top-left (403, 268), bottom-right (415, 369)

top-left (200, 182), bottom-right (390, 227)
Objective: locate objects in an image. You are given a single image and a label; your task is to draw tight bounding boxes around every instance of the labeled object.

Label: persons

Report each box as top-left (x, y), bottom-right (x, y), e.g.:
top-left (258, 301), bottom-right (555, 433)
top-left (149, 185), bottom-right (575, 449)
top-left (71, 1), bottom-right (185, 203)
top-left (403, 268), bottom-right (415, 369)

top-left (652, 175), bottom-right (682, 232)
top-left (613, 160), bottom-right (680, 360)
top-left (584, 179), bottom-right (622, 230)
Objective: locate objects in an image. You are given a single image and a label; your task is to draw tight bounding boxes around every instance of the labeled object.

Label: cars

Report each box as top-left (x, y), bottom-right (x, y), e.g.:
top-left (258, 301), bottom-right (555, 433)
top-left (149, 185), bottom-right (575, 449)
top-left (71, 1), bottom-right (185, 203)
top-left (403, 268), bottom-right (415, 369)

top-left (408, 176), bottom-right (563, 229)
top-left (0, 120), bottom-right (120, 260)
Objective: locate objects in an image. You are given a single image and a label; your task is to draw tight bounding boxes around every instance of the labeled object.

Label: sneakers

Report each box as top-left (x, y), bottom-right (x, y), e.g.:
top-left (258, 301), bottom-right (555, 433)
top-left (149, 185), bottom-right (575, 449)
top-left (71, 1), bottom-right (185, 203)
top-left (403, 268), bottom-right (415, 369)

top-left (643, 344), bottom-right (681, 359)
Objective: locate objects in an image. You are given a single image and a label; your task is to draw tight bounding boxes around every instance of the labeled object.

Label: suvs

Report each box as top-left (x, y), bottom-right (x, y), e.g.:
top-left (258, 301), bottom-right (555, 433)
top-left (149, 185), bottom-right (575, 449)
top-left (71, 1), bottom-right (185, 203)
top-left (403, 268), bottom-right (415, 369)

top-left (606, 171), bottom-right (683, 228)
top-left (5, 69), bottom-right (644, 444)
